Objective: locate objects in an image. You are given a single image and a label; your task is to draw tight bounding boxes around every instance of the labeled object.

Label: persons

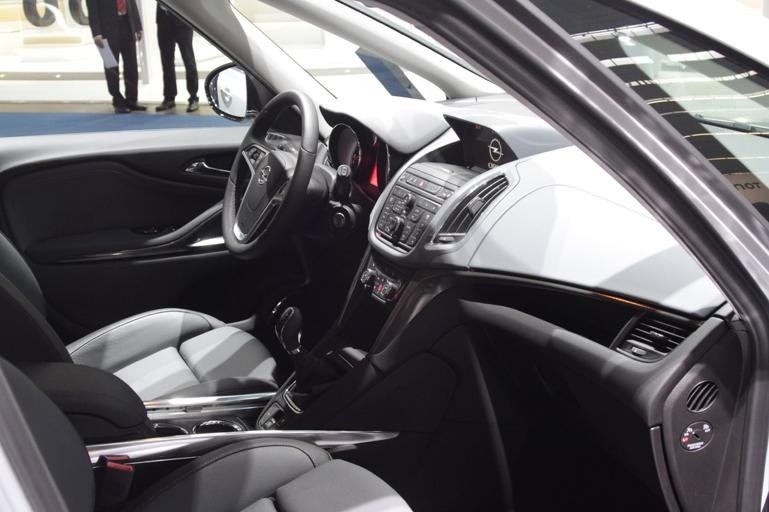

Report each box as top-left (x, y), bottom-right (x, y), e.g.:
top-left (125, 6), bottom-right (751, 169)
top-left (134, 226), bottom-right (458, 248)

top-left (156, 1), bottom-right (200, 112)
top-left (86, 0), bottom-right (147, 114)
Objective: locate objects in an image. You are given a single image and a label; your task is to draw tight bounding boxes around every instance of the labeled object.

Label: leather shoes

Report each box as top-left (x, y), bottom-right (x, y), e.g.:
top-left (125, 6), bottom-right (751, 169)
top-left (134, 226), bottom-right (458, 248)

top-left (155, 101), bottom-right (175, 111)
top-left (187, 100), bottom-right (198, 112)
top-left (114, 107), bottom-right (130, 113)
top-left (128, 105), bottom-right (146, 110)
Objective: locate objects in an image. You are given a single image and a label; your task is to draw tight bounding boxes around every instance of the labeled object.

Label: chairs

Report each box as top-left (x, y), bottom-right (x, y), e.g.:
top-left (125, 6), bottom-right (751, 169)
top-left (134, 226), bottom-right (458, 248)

top-left (0, 214), bottom-right (286, 422)
top-left (0, 351), bottom-right (423, 510)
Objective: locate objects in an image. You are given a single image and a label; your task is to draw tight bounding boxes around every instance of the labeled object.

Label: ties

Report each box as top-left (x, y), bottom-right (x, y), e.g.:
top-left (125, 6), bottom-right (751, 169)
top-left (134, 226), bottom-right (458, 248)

top-left (116, 0), bottom-right (126, 12)
